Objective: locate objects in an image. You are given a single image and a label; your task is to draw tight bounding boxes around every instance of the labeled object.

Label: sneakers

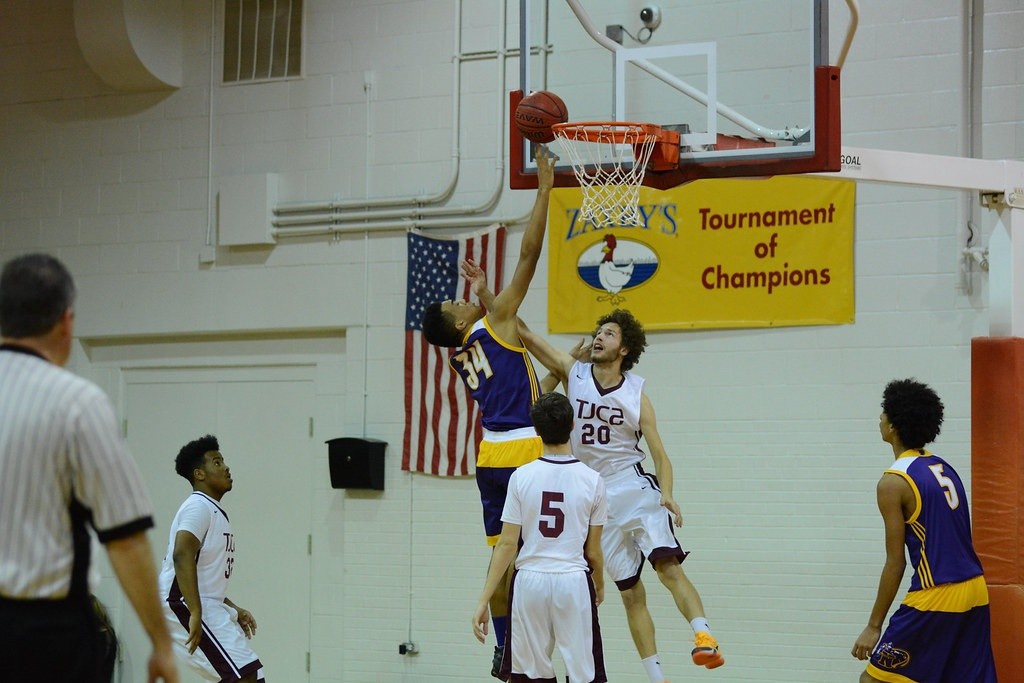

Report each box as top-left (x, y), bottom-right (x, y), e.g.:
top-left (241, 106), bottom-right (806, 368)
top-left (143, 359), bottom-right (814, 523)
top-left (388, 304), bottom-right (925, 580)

top-left (691, 632), bottom-right (724, 669)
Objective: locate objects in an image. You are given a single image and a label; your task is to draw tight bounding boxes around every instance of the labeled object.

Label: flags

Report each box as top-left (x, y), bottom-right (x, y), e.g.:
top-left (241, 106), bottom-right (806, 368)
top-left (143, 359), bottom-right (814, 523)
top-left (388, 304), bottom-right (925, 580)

top-left (401, 224), bottom-right (506, 477)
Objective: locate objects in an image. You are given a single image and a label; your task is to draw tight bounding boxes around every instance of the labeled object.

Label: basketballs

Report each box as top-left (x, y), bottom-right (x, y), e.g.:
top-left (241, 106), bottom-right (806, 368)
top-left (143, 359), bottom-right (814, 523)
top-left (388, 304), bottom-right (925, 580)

top-left (515, 90), bottom-right (569, 143)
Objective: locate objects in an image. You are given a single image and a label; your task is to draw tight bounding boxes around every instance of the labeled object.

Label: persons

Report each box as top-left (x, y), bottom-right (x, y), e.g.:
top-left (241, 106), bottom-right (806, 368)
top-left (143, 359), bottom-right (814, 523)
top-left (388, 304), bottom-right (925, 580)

top-left (0, 255), bottom-right (179, 683)
top-left (472, 392), bottom-right (608, 683)
top-left (422, 143), bottom-right (592, 683)
top-left (851, 378), bottom-right (997, 683)
top-left (159, 435), bottom-right (265, 683)
top-left (460, 259), bottom-right (725, 683)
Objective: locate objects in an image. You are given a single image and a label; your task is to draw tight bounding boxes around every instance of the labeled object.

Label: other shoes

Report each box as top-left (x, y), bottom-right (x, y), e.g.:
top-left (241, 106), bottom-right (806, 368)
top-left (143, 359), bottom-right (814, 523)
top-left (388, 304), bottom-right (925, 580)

top-left (491, 645), bottom-right (504, 670)
